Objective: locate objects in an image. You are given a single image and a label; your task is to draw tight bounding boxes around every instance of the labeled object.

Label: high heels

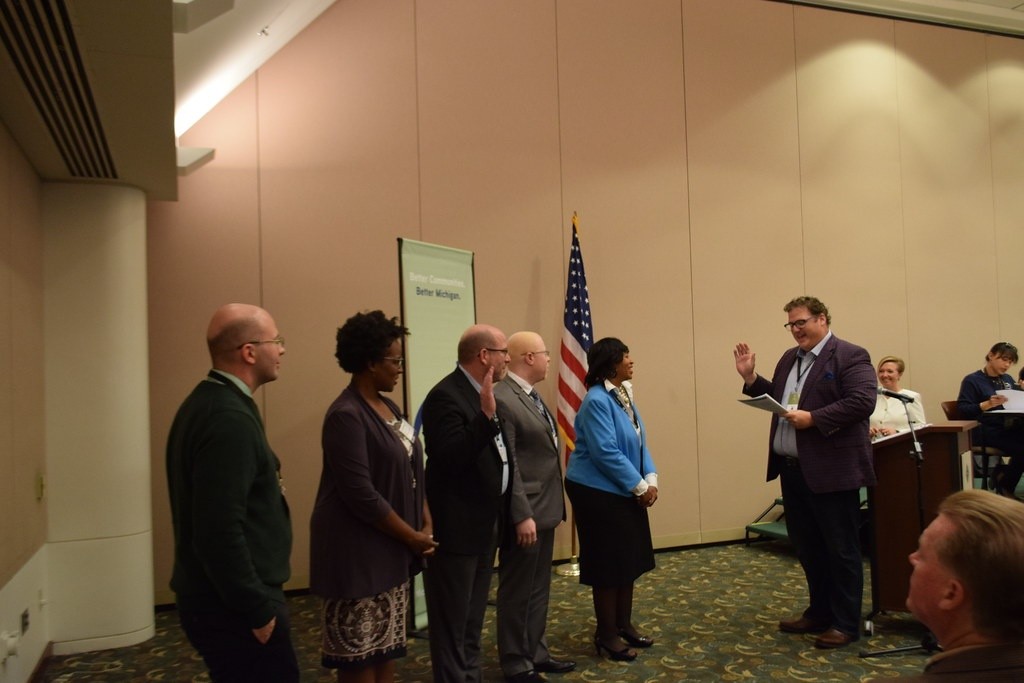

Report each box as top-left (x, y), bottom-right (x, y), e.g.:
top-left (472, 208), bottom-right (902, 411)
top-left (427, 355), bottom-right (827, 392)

top-left (594, 629), bottom-right (637, 661)
top-left (616, 623), bottom-right (653, 648)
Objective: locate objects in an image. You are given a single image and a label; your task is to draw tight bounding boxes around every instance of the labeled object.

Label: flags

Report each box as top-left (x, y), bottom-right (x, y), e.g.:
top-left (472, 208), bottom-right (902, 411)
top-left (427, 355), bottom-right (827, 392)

top-left (556, 218), bottom-right (595, 533)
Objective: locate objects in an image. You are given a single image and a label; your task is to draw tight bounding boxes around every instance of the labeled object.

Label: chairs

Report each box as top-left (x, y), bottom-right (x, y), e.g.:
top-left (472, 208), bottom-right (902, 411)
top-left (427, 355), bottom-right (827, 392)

top-left (941, 402), bottom-right (1012, 495)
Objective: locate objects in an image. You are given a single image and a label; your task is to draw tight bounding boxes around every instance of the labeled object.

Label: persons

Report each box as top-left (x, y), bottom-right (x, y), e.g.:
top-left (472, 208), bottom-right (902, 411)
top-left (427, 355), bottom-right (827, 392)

top-left (564, 336), bottom-right (658, 662)
top-left (420, 324), bottom-right (515, 683)
top-left (309, 310), bottom-right (440, 683)
top-left (734, 297), bottom-right (876, 650)
top-left (954, 342), bottom-right (1024, 498)
top-left (493, 331), bottom-right (578, 683)
top-left (869, 355), bottom-right (927, 441)
top-left (168, 302), bottom-right (299, 683)
top-left (867, 489), bottom-right (1024, 683)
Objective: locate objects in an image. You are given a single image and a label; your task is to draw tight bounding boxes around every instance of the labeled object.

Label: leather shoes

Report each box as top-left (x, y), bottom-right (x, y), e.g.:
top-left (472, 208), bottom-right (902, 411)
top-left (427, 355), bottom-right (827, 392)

top-left (534, 656), bottom-right (577, 673)
top-left (779, 614), bottom-right (826, 634)
top-left (815, 625), bottom-right (856, 649)
top-left (506, 669), bottom-right (550, 683)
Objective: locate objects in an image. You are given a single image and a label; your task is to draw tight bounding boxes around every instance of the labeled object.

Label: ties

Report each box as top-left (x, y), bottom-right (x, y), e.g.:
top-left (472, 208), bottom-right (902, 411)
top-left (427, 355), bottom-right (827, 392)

top-left (529, 388), bottom-right (546, 422)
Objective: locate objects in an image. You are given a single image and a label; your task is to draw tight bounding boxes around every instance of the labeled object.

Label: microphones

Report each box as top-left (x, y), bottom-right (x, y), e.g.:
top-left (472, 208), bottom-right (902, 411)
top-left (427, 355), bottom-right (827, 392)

top-left (877, 386), bottom-right (915, 403)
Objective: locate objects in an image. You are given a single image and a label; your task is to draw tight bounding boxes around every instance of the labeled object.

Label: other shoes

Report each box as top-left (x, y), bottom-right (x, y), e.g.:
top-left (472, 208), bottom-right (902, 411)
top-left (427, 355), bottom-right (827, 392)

top-left (990, 464), bottom-right (1022, 503)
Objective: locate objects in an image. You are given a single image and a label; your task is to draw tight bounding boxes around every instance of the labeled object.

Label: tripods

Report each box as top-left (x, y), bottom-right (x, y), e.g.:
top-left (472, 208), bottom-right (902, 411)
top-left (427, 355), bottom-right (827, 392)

top-left (859, 399), bottom-right (943, 658)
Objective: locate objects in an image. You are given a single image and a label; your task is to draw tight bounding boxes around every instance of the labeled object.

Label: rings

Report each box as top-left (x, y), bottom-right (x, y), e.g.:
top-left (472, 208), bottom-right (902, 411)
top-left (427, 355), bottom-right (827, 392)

top-left (884, 433), bottom-right (886, 435)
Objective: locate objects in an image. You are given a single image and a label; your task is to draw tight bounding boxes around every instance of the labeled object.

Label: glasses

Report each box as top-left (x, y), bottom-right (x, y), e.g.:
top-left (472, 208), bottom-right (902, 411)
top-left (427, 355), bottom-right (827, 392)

top-left (477, 346), bottom-right (507, 359)
top-left (236, 337), bottom-right (285, 352)
top-left (382, 355), bottom-right (404, 368)
top-left (784, 315), bottom-right (816, 331)
top-left (521, 349), bottom-right (550, 358)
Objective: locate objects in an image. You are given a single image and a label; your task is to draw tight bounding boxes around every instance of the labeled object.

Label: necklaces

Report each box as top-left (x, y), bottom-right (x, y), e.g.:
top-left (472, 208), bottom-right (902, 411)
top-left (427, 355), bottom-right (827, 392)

top-left (618, 386), bottom-right (628, 403)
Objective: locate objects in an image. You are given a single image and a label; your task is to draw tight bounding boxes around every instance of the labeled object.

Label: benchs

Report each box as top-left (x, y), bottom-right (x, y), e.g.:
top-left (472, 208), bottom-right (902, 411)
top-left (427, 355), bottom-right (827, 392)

top-left (744, 485), bottom-right (870, 550)
